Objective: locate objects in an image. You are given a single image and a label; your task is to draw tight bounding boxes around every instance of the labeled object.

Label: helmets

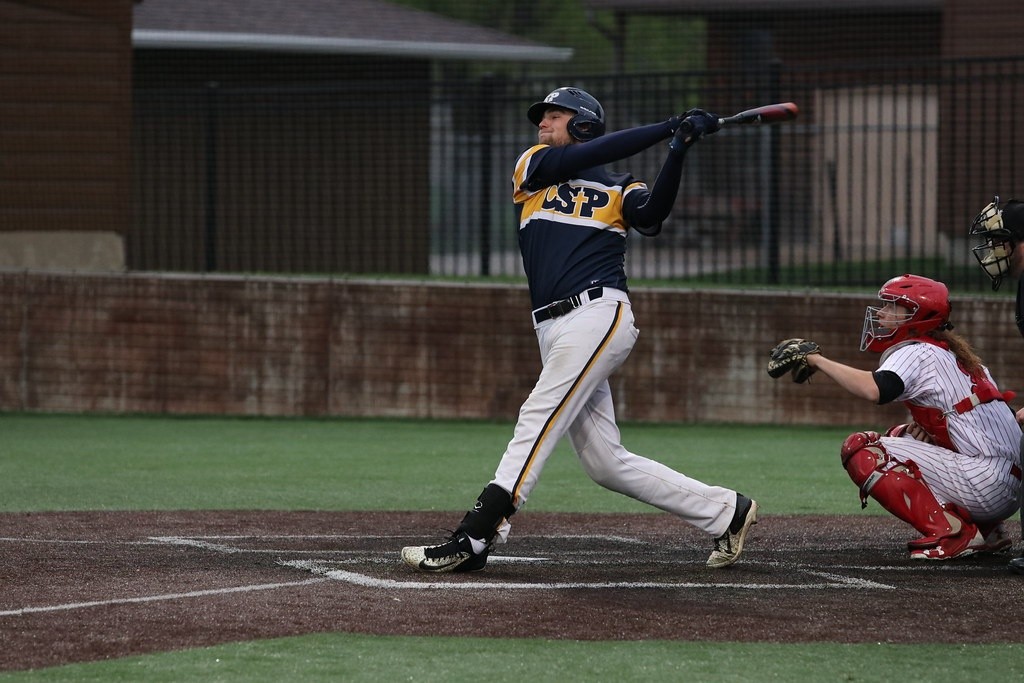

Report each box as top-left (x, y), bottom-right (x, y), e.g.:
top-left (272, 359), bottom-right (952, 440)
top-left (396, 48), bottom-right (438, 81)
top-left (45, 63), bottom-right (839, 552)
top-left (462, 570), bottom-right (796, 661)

top-left (526, 86), bottom-right (605, 143)
top-left (859, 274), bottom-right (951, 352)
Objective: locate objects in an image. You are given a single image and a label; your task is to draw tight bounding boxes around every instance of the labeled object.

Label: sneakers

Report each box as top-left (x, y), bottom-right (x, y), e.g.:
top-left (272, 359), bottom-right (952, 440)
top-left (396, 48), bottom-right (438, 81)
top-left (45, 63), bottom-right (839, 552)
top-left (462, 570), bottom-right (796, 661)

top-left (910, 523), bottom-right (988, 560)
top-left (982, 524), bottom-right (1013, 552)
top-left (401, 528), bottom-right (493, 573)
top-left (705, 492), bottom-right (758, 569)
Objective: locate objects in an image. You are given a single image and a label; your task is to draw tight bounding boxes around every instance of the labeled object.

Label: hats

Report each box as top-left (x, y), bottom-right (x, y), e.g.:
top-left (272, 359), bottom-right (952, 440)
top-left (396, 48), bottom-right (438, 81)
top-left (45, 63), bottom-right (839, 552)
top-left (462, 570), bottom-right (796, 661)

top-left (981, 198), bottom-right (1024, 238)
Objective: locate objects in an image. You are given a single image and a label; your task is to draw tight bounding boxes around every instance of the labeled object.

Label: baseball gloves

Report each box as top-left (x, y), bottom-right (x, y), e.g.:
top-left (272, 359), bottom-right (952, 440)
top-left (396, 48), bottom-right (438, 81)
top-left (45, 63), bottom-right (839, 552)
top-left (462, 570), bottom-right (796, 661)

top-left (766, 338), bottom-right (822, 385)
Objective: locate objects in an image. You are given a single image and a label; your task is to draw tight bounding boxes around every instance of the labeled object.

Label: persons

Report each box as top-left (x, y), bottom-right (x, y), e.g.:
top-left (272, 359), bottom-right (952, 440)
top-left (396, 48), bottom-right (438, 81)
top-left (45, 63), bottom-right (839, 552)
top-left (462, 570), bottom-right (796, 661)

top-left (400, 87), bottom-right (759, 574)
top-left (969, 198), bottom-right (1024, 568)
top-left (767, 274), bottom-right (1022, 559)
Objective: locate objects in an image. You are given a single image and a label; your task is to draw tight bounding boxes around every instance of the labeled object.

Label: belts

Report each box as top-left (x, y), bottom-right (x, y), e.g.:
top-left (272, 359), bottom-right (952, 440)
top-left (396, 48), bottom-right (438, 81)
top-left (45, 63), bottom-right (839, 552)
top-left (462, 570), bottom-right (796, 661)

top-left (534, 287), bottom-right (603, 324)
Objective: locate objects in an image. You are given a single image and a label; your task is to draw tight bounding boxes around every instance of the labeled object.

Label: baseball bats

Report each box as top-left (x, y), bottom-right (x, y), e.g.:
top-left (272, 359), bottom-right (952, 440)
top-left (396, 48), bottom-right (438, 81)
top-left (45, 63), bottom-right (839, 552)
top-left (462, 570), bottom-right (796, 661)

top-left (677, 101), bottom-right (800, 134)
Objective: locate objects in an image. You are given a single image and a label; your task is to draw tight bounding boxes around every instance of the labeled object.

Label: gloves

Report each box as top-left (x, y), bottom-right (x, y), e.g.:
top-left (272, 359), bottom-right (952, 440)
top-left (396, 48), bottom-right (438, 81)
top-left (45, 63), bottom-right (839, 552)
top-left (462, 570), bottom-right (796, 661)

top-left (668, 110), bottom-right (718, 154)
